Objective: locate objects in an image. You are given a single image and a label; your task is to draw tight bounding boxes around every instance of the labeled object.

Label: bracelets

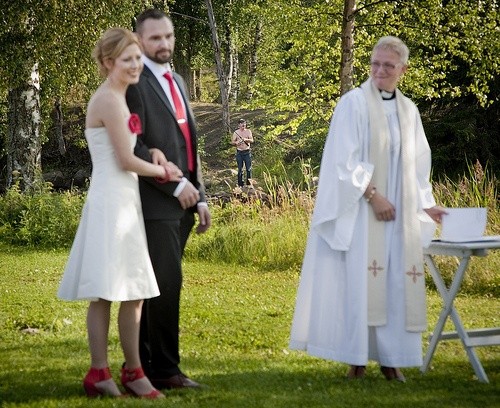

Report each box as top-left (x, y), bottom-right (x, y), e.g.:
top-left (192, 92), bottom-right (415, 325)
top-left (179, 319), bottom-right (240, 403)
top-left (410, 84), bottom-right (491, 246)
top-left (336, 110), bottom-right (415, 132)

top-left (365, 185), bottom-right (377, 206)
top-left (156, 165), bottom-right (170, 186)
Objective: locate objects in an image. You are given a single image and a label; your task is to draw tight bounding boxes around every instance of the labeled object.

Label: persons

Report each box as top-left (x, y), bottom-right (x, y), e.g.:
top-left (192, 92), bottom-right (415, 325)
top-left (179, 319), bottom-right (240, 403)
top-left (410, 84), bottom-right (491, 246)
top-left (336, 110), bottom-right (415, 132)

top-left (56, 27), bottom-right (183, 398)
top-left (230, 118), bottom-right (255, 186)
top-left (126, 7), bottom-right (212, 392)
top-left (289, 35), bottom-right (450, 385)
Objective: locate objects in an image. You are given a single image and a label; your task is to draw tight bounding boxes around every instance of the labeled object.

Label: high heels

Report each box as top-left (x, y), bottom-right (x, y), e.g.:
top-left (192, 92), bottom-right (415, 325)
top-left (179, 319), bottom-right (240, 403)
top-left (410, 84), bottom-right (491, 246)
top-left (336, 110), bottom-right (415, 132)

top-left (380, 364), bottom-right (405, 384)
top-left (348, 365), bottom-right (366, 379)
top-left (84, 367), bottom-right (123, 398)
top-left (120, 361), bottom-right (166, 401)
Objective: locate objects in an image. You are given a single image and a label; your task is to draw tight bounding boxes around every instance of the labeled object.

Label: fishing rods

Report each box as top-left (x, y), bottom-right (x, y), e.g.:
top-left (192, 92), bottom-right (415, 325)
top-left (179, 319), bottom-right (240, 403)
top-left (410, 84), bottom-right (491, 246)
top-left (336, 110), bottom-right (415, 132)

top-left (214, 110), bottom-right (249, 146)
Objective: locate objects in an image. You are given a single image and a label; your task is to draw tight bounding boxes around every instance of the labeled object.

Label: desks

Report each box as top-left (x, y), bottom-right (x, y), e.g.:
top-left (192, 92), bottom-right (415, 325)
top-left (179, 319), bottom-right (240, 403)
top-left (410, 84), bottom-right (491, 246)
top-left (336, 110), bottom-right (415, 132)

top-left (419, 237), bottom-right (500, 384)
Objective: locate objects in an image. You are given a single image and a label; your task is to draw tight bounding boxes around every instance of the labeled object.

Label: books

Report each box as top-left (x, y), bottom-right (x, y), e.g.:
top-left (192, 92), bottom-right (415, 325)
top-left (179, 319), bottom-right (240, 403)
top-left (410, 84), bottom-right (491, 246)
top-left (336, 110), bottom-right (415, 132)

top-left (441, 207), bottom-right (500, 243)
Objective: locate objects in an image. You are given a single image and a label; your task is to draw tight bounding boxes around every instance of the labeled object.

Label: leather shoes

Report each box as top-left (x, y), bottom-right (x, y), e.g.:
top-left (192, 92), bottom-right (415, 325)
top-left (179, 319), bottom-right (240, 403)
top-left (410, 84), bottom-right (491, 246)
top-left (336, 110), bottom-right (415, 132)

top-left (162, 373), bottom-right (201, 388)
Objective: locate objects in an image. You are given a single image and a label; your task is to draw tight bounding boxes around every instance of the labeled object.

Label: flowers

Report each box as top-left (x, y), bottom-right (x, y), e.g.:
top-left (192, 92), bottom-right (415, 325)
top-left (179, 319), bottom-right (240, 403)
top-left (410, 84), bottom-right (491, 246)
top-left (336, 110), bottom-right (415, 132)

top-left (129, 113), bottom-right (142, 135)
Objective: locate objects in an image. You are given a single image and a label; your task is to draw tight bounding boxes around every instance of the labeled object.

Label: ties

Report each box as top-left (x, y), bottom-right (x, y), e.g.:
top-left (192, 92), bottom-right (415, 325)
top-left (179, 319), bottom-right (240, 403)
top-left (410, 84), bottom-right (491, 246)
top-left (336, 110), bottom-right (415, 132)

top-left (163, 71), bottom-right (194, 171)
